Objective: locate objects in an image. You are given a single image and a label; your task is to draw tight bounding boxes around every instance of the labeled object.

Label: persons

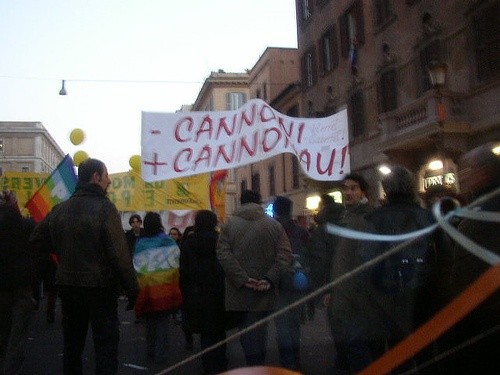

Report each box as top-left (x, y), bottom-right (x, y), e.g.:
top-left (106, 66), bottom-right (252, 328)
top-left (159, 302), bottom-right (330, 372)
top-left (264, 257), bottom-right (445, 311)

top-left (0, 190), bottom-right (34, 375)
top-left (30, 159), bottom-right (140, 375)
top-left (19, 205), bottom-right (60, 324)
top-left (120, 142), bottom-right (500, 375)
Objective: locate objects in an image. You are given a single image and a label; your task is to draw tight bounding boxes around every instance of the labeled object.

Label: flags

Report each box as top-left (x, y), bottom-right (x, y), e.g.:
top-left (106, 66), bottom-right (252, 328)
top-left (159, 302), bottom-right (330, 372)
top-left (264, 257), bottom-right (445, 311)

top-left (27, 154), bottom-right (78, 225)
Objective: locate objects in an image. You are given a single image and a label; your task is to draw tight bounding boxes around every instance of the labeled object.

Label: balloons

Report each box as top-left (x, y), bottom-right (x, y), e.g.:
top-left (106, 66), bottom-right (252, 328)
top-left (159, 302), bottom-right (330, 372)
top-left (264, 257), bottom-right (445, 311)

top-left (70, 128), bottom-right (91, 167)
top-left (129, 155), bottom-right (141, 169)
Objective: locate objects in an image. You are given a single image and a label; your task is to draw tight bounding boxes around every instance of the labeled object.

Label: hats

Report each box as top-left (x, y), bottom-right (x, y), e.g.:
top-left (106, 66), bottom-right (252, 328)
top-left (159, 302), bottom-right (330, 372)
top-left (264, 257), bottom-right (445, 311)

top-left (382, 166), bottom-right (417, 201)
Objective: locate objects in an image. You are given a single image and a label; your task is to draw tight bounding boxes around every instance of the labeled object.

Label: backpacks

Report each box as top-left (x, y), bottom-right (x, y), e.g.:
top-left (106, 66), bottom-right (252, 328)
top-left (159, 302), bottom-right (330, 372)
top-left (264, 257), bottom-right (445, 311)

top-left (282, 240), bottom-right (312, 290)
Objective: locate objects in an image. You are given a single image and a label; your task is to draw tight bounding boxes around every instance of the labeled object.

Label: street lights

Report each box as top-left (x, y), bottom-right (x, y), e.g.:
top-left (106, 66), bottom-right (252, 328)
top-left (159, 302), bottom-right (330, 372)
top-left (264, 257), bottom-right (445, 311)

top-left (427, 60), bottom-right (447, 185)
